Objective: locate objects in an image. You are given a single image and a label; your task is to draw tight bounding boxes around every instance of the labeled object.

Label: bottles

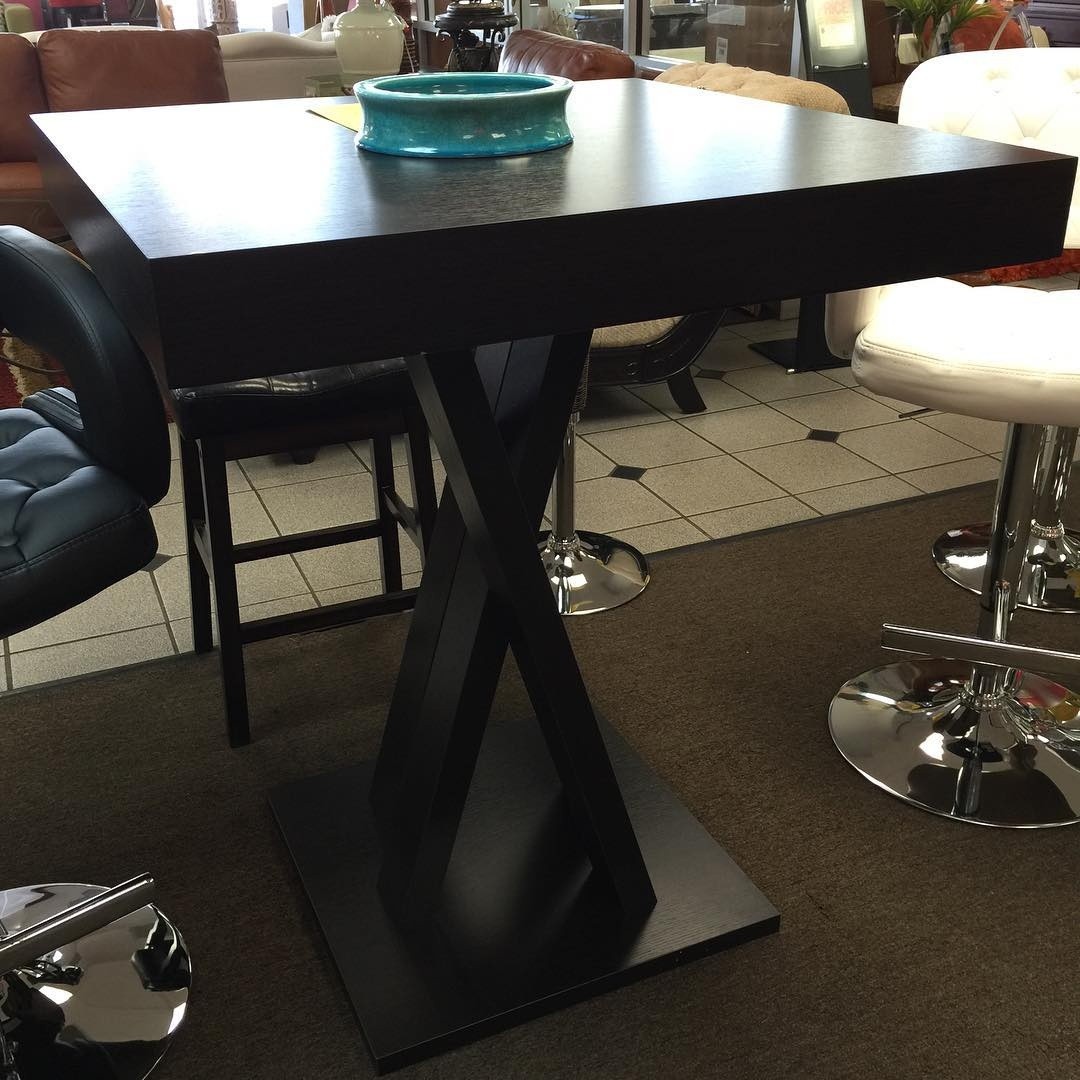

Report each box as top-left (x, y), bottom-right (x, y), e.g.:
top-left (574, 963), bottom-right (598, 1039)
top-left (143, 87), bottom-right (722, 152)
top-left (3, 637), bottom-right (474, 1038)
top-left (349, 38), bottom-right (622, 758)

top-left (333, 0), bottom-right (404, 88)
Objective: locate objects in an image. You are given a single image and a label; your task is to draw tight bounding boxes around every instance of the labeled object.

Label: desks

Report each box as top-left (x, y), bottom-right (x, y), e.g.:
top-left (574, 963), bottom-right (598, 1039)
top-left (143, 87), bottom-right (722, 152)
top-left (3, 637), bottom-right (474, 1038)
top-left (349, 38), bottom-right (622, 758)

top-left (28, 73), bottom-right (1080, 1065)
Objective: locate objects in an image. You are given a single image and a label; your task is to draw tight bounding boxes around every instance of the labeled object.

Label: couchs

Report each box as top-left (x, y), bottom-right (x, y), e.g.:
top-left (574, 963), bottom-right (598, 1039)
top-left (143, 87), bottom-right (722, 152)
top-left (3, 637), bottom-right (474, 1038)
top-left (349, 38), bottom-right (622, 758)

top-left (0, 0), bottom-right (852, 417)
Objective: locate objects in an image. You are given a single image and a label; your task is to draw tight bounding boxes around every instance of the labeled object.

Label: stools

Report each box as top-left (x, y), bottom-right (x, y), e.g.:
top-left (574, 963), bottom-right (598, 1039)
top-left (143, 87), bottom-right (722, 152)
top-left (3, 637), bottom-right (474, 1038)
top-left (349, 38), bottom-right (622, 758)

top-left (0, 221), bottom-right (194, 1080)
top-left (163, 355), bottom-right (436, 747)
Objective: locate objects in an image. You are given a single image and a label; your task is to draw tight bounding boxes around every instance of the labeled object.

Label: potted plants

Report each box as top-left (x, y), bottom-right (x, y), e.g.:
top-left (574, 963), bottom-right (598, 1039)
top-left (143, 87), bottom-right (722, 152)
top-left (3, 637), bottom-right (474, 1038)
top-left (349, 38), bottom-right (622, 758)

top-left (884, 0), bottom-right (995, 81)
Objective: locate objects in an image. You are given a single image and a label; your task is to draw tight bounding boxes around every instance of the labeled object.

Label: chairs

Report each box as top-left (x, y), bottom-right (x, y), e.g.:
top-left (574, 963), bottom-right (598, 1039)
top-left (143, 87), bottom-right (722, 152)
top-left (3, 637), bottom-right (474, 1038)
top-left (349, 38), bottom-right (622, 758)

top-left (824, 40), bottom-right (1079, 827)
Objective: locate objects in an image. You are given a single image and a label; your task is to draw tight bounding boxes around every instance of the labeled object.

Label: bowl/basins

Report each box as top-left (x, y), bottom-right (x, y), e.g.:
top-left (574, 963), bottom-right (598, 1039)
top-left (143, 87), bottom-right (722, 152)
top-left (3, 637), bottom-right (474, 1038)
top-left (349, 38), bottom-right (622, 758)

top-left (352, 70), bottom-right (574, 157)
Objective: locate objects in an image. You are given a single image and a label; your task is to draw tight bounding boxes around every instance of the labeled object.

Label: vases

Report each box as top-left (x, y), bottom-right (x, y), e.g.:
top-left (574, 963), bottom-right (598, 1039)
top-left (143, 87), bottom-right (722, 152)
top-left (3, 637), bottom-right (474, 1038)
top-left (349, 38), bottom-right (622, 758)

top-left (332, 1), bottom-right (404, 86)
